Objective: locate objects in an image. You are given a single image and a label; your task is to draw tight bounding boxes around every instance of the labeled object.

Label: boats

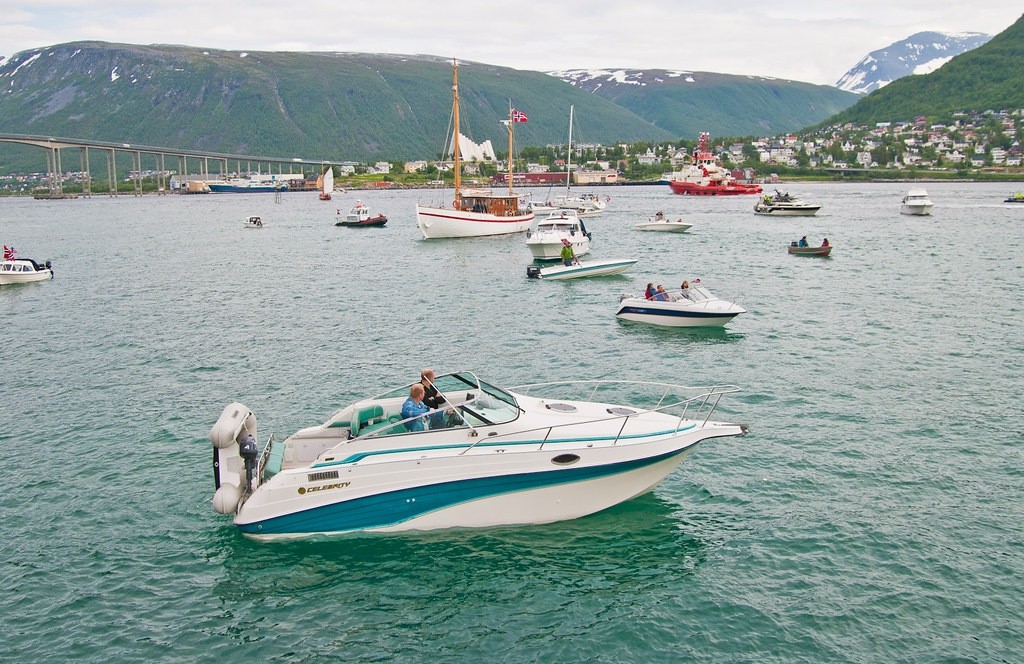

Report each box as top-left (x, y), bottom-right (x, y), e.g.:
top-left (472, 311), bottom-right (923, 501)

top-left (750, 190), bottom-right (824, 217)
top-left (243, 215), bottom-right (263, 228)
top-left (657, 128), bottom-right (764, 197)
top-left (899, 187), bottom-right (934, 216)
top-left (614, 281), bottom-right (748, 330)
top-left (525, 257), bottom-right (640, 281)
top-left (334, 198), bottom-right (388, 228)
top-left (178, 154), bottom-right (323, 195)
top-left (206, 368), bottom-right (750, 545)
top-left (787, 240), bottom-right (833, 257)
top-left (526, 206), bottom-right (593, 262)
top-left (1004, 191), bottom-right (1024, 203)
top-left (634, 213), bottom-right (694, 234)
top-left (0, 257), bottom-right (55, 287)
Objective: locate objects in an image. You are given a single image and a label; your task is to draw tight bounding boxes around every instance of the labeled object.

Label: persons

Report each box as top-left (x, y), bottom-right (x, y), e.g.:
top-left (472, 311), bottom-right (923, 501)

top-left (799, 236), bottom-right (809, 247)
top-left (417, 369), bottom-right (447, 409)
top-left (561, 241), bottom-right (576, 266)
top-left (820, 237), bottom-right (830, 247)
top-left (400, 383), bottom-right (458, 432)
top-left (646, 279), bottom-right (702, 302)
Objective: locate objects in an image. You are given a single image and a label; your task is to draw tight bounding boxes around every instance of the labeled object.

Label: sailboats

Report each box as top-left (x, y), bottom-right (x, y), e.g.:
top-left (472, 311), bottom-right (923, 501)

top-left (414, 57), bottom-right (539, 240)
top-left (527, 101), bottom-right (612, 219)
top-left (318, 165), bottom-right (334, 201)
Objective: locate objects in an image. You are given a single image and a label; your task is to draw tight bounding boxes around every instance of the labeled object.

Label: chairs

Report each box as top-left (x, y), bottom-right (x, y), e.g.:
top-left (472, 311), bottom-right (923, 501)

top-left (387, 411), bottom-right (408, 433)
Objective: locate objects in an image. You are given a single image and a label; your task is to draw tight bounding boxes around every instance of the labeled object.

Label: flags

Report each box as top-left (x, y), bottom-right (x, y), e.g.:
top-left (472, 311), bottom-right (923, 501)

top-left (512, 109), bottom-right (529, 123)
top-left (3, 245), bottom-right (15, 261)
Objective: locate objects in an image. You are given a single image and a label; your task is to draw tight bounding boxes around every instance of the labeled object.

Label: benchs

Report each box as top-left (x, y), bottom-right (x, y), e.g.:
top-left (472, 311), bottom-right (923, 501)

top-left (350, 406), bottom-right (393, 437)
